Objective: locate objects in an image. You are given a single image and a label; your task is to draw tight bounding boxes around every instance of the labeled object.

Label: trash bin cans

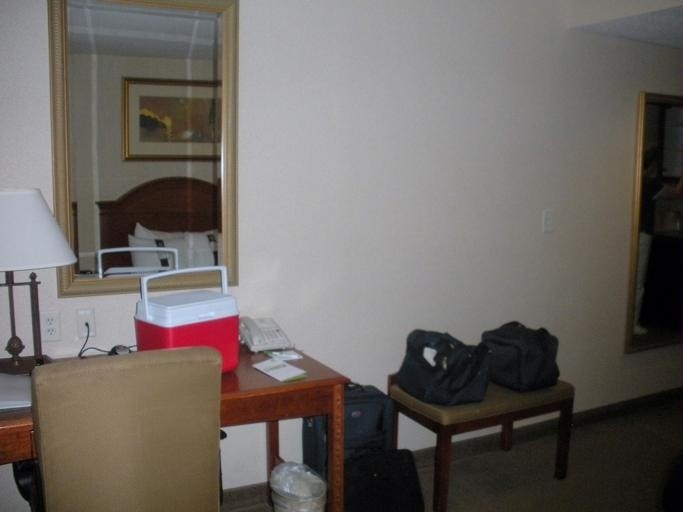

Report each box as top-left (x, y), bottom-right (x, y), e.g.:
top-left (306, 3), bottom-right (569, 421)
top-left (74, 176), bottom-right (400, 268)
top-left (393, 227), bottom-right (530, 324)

top-left (270, 462), bottom-right (327, 512)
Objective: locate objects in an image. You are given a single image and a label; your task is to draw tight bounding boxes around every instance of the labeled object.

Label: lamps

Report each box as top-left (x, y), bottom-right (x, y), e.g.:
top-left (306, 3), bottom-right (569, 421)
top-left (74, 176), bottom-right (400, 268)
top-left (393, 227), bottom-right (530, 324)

top-left (0, 188), bottom-right (81, 375)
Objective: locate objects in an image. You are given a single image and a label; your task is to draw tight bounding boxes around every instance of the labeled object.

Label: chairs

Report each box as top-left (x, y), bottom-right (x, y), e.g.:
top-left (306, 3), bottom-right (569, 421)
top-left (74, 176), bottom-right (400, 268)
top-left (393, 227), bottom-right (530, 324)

top-left (12, 346), bottom-right (229, 512)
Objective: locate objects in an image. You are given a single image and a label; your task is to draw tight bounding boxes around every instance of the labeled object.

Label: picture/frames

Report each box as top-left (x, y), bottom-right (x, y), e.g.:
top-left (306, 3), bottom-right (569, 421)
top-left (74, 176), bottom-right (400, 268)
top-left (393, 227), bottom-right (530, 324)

top-left (122, 78), bottom-right (224, 161)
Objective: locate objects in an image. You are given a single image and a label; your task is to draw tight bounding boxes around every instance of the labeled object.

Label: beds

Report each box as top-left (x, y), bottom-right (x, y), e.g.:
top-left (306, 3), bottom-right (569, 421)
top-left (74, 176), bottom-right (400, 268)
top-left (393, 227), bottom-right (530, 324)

top-left (94, 176), bottom-right (221, 278)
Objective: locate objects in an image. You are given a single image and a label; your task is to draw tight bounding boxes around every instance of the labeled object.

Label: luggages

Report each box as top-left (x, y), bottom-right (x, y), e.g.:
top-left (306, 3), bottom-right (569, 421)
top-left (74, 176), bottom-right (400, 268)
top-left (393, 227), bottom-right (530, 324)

top-left (301, 379), bottom-right (399, 484)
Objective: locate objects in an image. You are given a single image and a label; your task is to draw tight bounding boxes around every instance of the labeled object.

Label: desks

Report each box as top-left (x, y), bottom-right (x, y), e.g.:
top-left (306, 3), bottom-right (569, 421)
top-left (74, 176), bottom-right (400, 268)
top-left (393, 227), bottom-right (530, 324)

top-left (0, 344), bottom-right (351, 512)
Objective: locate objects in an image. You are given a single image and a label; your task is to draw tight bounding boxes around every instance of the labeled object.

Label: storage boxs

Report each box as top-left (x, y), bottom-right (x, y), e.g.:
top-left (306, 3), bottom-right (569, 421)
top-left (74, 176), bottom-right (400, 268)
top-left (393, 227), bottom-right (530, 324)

top-left (130, 265), bottom-right (242, 375)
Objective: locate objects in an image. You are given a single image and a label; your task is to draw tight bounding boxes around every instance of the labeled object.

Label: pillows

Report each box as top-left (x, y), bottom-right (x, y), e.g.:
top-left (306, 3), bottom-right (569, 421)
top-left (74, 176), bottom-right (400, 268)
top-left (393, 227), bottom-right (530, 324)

top-left (126, 220), bottom-right (222, 272)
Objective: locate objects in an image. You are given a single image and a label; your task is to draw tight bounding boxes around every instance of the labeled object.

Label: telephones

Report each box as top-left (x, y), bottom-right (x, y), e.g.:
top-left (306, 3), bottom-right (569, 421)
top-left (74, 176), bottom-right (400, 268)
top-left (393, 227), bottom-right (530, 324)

top-left (239, 316), bottom-right (293, 352)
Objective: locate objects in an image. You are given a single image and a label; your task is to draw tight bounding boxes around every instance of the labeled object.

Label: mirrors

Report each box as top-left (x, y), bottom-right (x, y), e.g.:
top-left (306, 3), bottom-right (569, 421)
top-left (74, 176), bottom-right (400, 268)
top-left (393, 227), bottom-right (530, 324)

top-left (46, 1), bottom-right (240, 299)
top-left (626, 89), bottom-right (683, 354)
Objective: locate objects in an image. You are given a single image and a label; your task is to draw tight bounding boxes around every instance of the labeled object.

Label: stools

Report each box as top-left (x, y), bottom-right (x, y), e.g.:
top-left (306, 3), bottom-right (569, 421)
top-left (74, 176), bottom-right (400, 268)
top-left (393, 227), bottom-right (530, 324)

top-left (386, 372), bottom-right (574, 512)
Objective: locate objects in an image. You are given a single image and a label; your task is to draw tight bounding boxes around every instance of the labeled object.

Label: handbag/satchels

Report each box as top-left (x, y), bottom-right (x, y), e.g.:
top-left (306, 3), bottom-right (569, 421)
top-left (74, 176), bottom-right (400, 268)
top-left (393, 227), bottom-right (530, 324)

top-left (483, 322), bottom-right (560, 393)
top-left (343, 448), bottom-right (426, 512)
top-left (395, 327), bottom-right (488, 407)
